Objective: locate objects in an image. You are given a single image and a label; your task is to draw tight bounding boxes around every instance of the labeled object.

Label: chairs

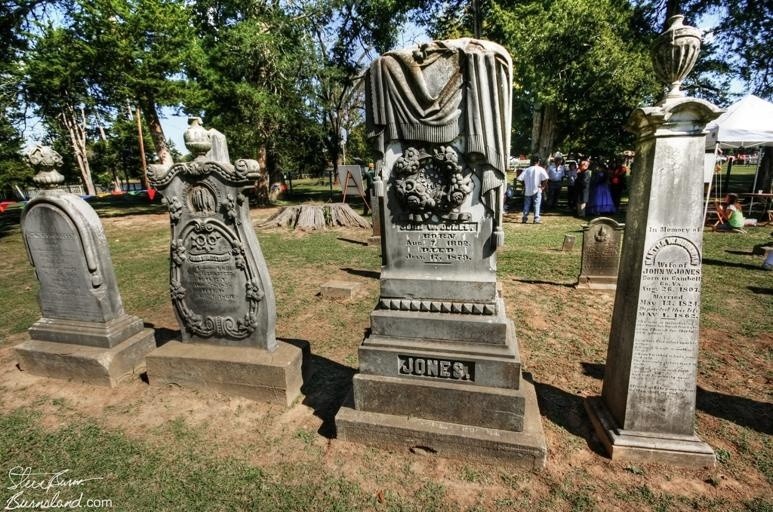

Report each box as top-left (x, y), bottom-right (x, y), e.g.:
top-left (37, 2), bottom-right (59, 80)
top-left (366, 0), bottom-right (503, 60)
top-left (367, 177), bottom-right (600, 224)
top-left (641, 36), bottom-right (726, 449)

top-left (507, 178), bottom-right (525, 213)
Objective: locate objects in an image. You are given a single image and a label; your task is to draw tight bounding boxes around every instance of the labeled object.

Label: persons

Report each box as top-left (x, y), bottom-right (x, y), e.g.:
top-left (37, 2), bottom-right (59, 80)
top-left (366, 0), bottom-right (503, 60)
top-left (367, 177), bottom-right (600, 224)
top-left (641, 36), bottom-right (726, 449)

top-left (509, 145), bottom-right (630, 225)
top-left (710, 192), bottom-right (743, 232)
top-left (358, 161), bottom-right (375, 217)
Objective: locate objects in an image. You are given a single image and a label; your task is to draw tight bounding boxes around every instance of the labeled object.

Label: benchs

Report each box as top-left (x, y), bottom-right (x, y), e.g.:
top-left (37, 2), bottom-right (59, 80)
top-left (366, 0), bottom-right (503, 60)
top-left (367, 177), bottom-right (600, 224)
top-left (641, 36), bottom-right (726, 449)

top-left (704, 193), bottom-right (773, 231)
